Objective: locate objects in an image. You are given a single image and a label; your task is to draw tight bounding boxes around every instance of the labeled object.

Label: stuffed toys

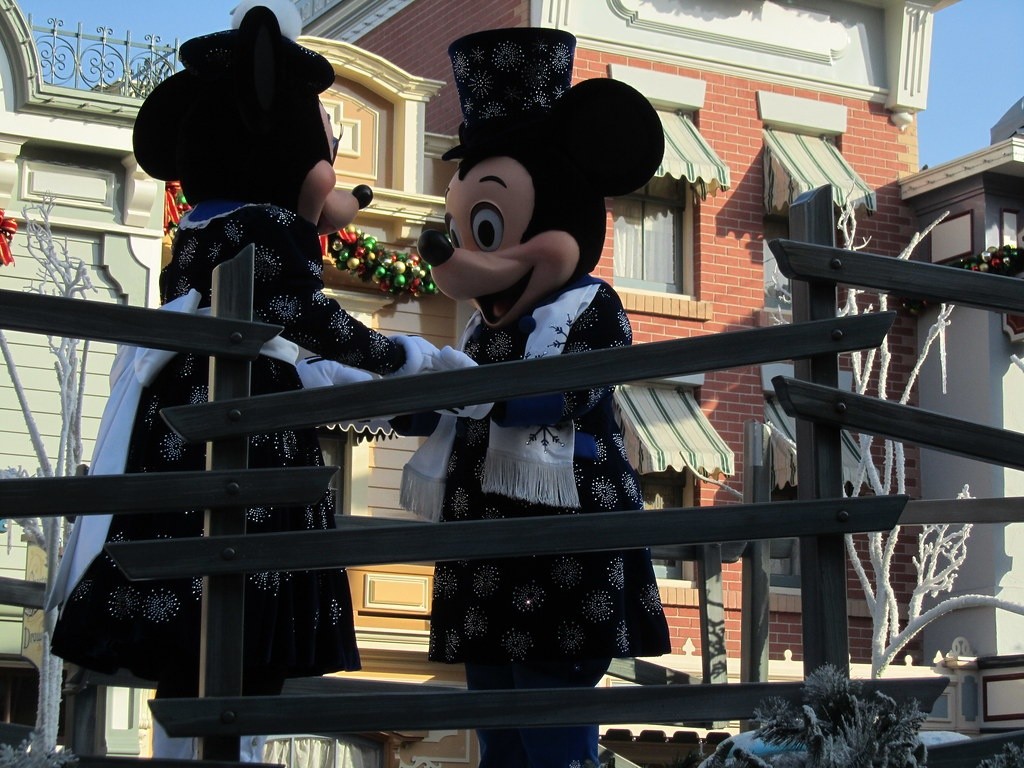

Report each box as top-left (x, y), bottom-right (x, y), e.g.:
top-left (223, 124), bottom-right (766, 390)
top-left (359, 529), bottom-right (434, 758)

top-left (51, 6), bottom-right (672, 767)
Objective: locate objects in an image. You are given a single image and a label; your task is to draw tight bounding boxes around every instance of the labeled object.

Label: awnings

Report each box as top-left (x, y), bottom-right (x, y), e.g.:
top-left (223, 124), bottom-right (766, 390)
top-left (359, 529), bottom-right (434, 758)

top-left (612, 381), bottom-right (735, 482)
top-left (765, 129), bottom-right (876, 214)
top-left (764, 398), bottom-right (879, 489)
top-left (654, 110), bottom-right (731, 201)
